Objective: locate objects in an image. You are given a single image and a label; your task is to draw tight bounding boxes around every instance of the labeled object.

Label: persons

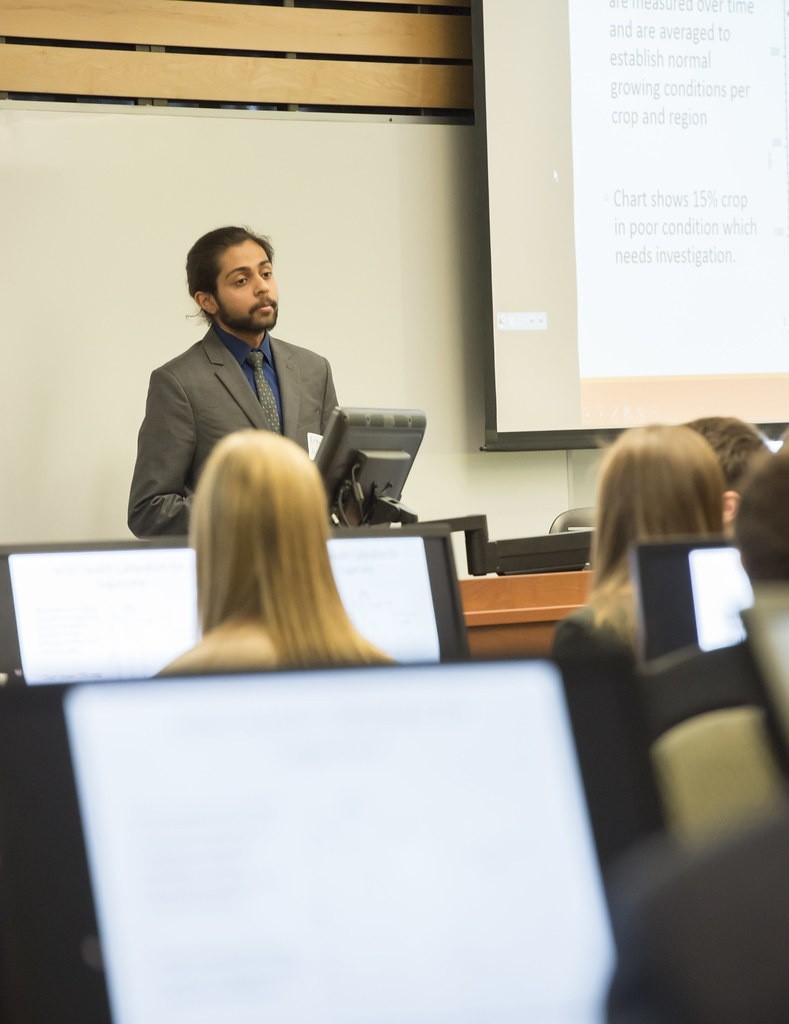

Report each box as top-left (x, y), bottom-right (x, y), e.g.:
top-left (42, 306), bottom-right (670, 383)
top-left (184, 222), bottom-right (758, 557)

top-left (544, 414), bottom-right (789, 1024)
top-left (149, 428), bottom-right (402, 679)
top-left (126, 226), bottom-right (342, 542)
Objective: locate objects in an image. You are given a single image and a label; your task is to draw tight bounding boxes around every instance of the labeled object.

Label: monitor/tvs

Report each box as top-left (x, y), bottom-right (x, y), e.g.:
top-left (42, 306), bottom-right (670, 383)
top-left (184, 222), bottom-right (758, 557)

top-left (312, 408), bottom-right (427, 527)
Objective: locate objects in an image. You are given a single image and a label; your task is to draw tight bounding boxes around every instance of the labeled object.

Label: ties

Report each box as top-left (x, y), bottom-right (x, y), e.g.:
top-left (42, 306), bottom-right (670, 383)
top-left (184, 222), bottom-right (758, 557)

top-left (247, 351), bottom-right (280, 438)
